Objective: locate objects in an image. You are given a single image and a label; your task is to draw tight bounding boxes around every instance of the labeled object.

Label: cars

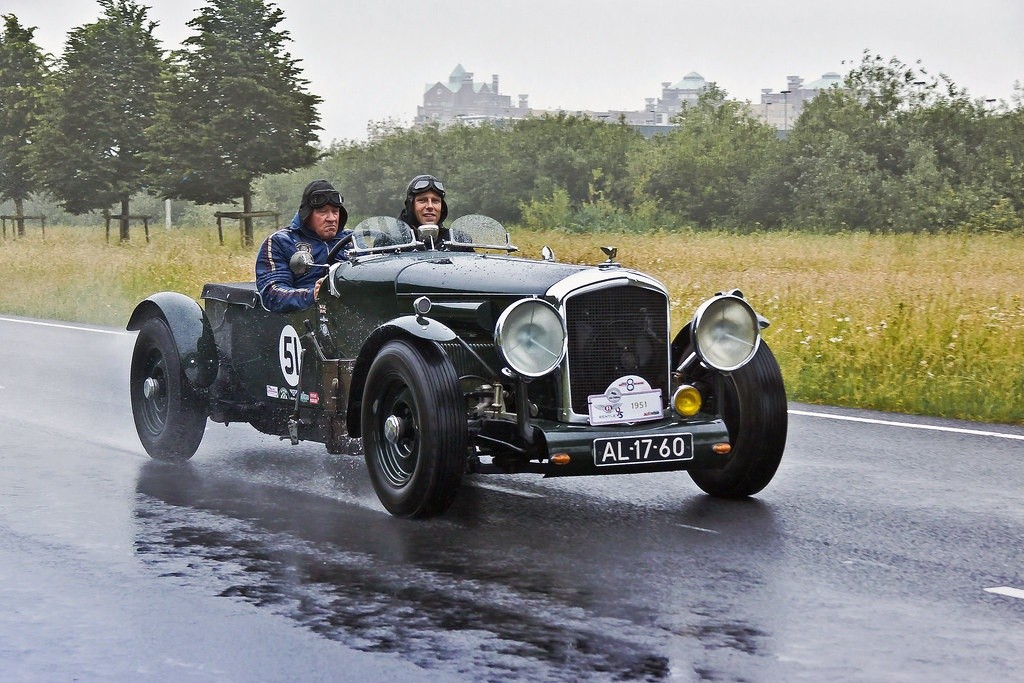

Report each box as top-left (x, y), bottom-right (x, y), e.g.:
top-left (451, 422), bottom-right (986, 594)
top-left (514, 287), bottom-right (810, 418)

top-left (126, 215), bottom-right (788, 519)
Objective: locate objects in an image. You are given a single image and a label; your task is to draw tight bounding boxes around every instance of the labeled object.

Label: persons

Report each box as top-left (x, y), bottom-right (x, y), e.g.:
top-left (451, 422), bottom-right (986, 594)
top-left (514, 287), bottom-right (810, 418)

top-left (255, 180), bottom-right (367, 311)
top-left (374, 174), bottom-right (473, 254)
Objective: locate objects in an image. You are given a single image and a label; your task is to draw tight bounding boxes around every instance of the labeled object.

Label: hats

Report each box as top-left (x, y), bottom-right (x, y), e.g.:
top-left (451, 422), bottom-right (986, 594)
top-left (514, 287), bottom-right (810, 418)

top-left (405, 174), bottom-right (448, 224)
top-left (298, 180), bottom-right (348, 234)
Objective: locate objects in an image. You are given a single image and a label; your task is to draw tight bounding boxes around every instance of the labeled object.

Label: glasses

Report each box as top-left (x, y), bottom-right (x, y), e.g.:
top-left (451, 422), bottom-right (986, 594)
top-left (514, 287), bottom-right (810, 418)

top-left (413, 178), bottom-right (445, 192)
top-left (308, 189), bottom-right (344, 208)
